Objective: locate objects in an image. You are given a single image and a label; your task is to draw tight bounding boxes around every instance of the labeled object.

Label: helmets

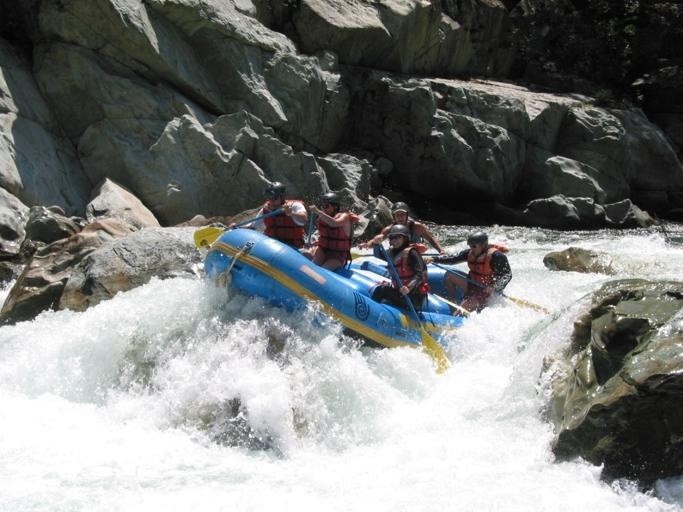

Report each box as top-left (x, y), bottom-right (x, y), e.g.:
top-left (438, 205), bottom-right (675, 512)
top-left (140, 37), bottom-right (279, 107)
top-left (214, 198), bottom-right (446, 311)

top-left (265, 181), bottom-right (285, 199)
top-left (467, 232), bottom-right (487, 245)
top-left (389, 224), bottom-right (409, 237)
top-left (391, 202), bottom-right (409, 214)
top-left (321, 193), bottom-right (340, 207)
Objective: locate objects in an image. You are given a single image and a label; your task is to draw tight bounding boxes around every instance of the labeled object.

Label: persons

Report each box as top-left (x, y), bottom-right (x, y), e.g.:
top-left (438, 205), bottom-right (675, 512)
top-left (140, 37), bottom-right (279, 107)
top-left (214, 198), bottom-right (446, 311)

top-left (357, 202), bottom-right (445, 257)
top-left (421, 231), bottom-right (512, 318)
top-left (307, 193), bottom-right (352, 272)
top-left (371, 224), bottom-right (428, 311)
top-left (227, 181), bottom-right (308, 250)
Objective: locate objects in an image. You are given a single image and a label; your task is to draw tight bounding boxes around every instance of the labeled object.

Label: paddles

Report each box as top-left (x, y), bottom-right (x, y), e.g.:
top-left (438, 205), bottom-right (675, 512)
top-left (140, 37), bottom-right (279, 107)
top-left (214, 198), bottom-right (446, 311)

top-left (192, 204), bottom-right (291, 249)
top-left (351, 252), bottom-right (374, 259)
top-left (373, 235), bottom-right (450, 375)
top-left (431, 261), bottom-right (550, 318)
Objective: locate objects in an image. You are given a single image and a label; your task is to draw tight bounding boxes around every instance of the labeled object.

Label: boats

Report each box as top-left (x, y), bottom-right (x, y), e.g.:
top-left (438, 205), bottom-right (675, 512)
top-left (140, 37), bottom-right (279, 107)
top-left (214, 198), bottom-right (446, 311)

top-left (202, 227), bottom-right (495, 352)
